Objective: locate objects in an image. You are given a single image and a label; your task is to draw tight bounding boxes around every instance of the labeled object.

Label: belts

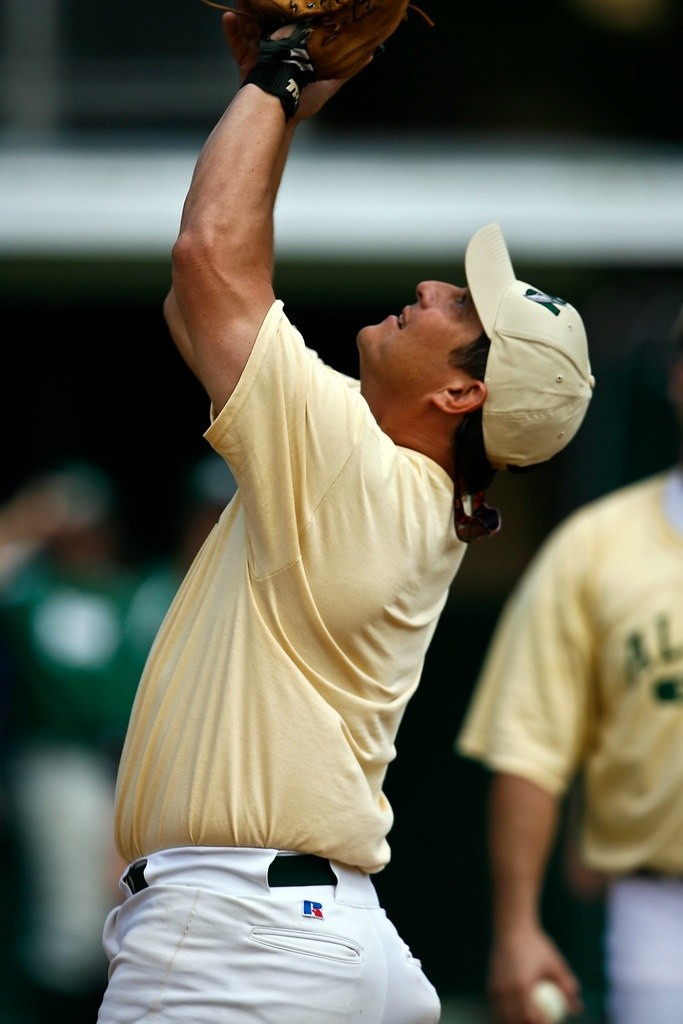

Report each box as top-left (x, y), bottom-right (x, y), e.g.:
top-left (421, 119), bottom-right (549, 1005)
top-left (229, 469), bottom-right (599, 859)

top-left (122, 853), bottom-right (338, 896)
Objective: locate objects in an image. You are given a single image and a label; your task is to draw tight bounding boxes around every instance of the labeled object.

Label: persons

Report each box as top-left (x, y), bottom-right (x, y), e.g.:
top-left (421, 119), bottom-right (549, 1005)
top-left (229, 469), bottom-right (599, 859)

top-left (0, 456), bottom-right (238, 1024)
top-left (458, 460), bottom-right (683, 1024)
top-left (97, 0), bottom-right (599, 1024)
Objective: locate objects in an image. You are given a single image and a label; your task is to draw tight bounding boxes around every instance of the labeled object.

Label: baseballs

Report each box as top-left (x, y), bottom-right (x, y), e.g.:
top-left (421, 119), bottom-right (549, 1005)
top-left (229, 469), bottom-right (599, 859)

top-left (530, 978), bottom-right (569, 1023)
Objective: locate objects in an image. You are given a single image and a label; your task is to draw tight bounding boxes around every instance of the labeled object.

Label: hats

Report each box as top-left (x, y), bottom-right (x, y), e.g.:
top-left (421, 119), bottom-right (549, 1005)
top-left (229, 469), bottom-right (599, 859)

top-left (465, 224), bottom-right (596, 473)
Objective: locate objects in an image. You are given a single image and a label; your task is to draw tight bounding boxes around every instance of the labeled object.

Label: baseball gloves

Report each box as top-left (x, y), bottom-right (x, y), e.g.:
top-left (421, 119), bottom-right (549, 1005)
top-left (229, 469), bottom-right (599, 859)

top-left (202, 0), bottom-right (438, 88)
